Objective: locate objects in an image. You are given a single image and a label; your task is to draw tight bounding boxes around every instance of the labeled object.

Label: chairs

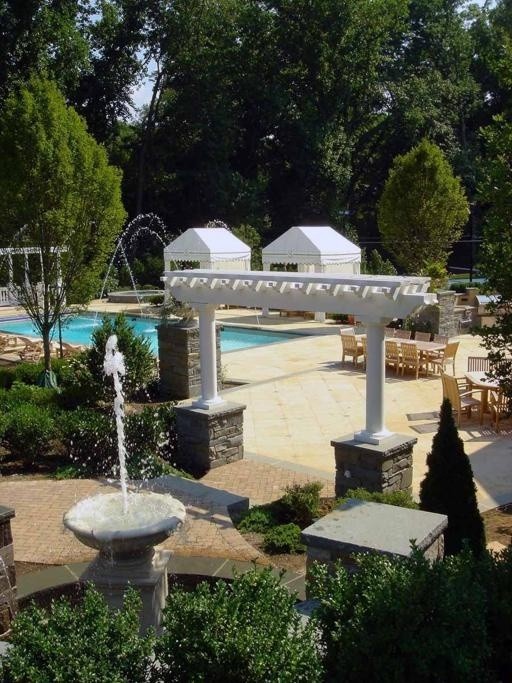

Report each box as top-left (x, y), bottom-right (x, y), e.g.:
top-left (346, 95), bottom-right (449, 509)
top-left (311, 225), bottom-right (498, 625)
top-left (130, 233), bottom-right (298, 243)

top-left (339, 325), bottom-right (460, 381)
top-left (439, 356), bottom-right (508, 432)
